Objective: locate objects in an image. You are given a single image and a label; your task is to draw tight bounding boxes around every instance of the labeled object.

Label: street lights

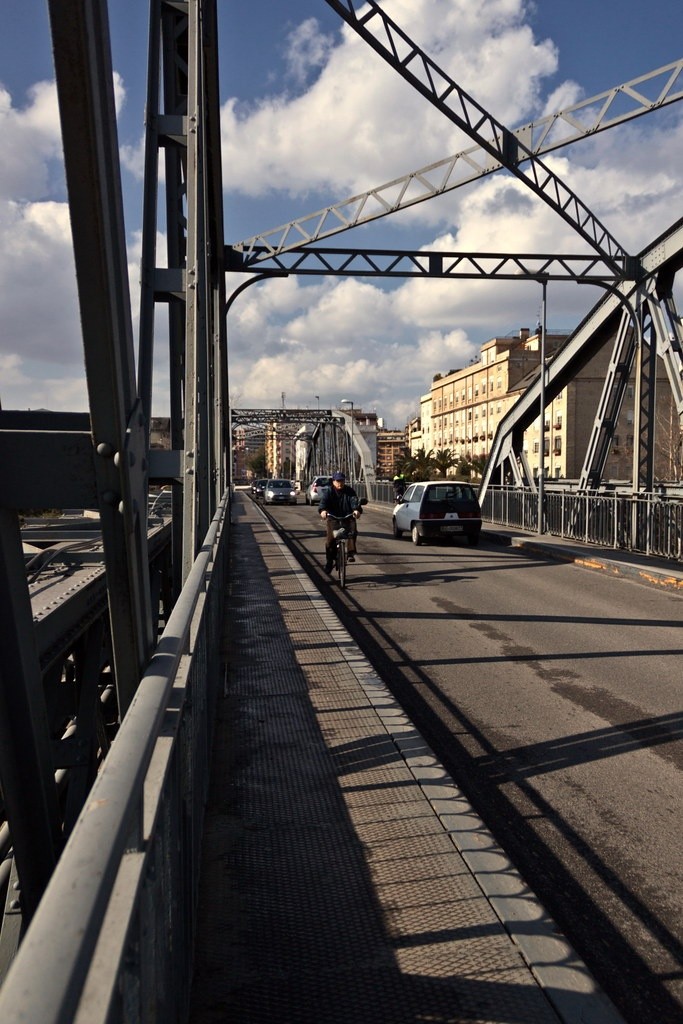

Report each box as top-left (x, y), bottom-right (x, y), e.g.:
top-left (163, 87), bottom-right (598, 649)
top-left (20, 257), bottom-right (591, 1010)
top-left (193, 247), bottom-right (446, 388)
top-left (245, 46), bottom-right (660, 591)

top-left (315, 395), bottom-right (320, 409)
top-left (341, 398), bottom-right (355, 489)
top-left (518, 267), bottom-right (547, 533)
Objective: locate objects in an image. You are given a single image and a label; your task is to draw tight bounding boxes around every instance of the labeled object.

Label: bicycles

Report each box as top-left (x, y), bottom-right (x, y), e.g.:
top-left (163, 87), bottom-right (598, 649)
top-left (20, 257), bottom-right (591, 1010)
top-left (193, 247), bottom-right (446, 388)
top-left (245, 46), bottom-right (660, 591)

top-left (327, 512), bottom-right (355, 588)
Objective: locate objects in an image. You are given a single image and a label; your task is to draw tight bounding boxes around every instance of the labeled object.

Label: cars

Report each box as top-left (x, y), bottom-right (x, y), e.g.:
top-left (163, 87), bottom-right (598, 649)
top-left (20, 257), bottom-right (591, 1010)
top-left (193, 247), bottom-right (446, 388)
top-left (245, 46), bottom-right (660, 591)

top-left (392, 481), bottom-right (483, 546)
top-left (306, 475), bottom-right (334, 506)
top-left (250, 479), bottom-right (268, 499)
top-left (264, 478), bottom-right (297, 504)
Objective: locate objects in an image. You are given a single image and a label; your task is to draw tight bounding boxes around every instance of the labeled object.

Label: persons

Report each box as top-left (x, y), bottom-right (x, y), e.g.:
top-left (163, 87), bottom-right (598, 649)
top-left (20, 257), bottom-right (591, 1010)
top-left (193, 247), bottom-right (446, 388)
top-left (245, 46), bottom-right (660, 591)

top-left (393, 469), bottom-right (407, 503)
top-left (317, 472), bottom-right (363, 574)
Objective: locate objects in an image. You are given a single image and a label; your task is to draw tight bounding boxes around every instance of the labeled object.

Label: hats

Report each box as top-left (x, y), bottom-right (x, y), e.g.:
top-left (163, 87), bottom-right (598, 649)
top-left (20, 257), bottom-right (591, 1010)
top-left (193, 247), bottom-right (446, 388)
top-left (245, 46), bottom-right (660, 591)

top-left (334, 473), bottom-right (345, 479)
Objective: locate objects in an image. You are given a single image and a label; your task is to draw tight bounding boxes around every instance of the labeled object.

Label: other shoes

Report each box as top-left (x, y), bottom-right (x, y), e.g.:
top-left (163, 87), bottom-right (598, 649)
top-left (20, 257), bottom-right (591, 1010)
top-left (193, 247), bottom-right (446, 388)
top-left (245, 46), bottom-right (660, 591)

top-left (325, 561), bottom-right (334, 574)
top-left (347, 554), bottom-right (355, 561)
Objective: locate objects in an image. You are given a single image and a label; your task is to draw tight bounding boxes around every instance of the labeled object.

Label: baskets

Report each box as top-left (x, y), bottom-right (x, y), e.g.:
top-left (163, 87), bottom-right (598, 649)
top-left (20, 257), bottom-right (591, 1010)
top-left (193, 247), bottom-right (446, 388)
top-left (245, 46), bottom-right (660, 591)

top-left (328, 519), bottom-right (356, 539)
